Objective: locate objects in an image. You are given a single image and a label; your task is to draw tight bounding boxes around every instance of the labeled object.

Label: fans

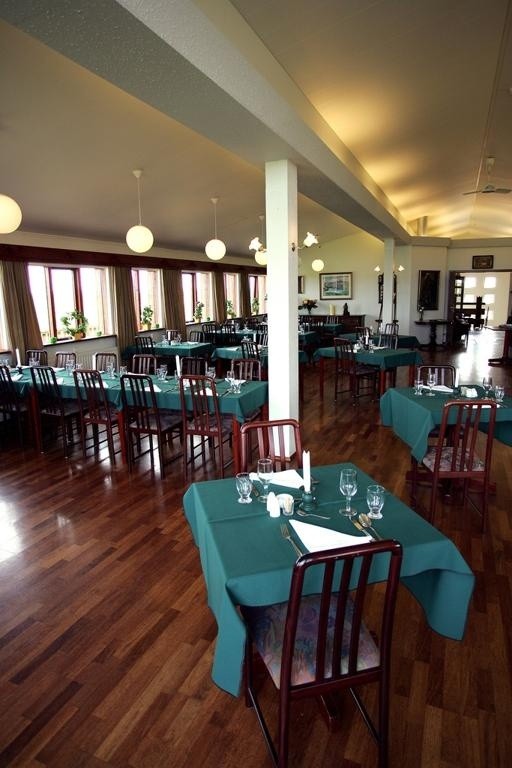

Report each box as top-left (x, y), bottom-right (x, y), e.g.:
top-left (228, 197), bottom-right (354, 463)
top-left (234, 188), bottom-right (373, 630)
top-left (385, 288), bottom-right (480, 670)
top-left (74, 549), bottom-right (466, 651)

top-left (461, 157), bottom-right (512, 195)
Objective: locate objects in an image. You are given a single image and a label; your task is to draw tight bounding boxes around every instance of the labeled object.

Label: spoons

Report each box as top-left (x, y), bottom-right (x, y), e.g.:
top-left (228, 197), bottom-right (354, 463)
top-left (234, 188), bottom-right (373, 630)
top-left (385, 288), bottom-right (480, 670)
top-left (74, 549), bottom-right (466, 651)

top-left (296, 510), bottom-right (330, 519)
top-left (359, 513), bottom-right (384, 540)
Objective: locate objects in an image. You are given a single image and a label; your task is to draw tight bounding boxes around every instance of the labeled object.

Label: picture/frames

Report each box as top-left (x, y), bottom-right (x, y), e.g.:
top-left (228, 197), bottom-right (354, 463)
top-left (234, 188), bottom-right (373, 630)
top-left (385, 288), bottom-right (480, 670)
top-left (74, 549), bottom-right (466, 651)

top-left (472, 255), bottom-right (494, 269)
top-left (320, 272), bottom-right (353, 300)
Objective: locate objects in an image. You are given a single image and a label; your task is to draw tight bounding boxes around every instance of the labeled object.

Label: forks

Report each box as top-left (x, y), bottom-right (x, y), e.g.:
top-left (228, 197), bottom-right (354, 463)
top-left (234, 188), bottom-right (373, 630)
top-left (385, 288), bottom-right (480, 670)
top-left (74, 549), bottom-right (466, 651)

top-left (280, 524), bottom-right (302, 557)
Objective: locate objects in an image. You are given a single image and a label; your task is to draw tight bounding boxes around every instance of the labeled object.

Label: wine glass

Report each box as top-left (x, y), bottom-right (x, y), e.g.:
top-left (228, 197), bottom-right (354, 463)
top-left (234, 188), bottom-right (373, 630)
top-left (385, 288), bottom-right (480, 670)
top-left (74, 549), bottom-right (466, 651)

top-left (226, 371), bottom-right (234, 390)
top-left (175, 370), bottom-right (181, 385)
top-left (427, 375), bottom-right (435, 397)
top-left (257, 459), bottom-right (272, 505)
top-left (495, 386), bottom-right (504, 402)
top-left (209, 367), bottom-right (216, 378)
top-left (482, 377), bottom-right (491, 400)
top-left (120, 366), bottom-right (127, 385)
top-left (106, 362), bottom-right (113, 374)
top-left (339, 469), bottom-right (358, 516)
top-left (369, 345), bottom-right (374, 353)
top-left (65, 365), bottom-right (72, 377)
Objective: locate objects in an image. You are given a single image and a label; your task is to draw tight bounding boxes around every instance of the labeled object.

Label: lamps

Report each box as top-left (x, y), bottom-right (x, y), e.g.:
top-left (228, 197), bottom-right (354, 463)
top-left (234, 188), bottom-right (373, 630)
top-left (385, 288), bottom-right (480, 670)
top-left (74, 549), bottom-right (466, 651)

top-left (393, 265), bottom-right (405, 335)
top-left (254, 215), bottom-right (267, 266)
top-left (374, 266), bottom-right (384, 333)
top-left (205, 198), bottom-right (227, 261)
top-left (292, 231), bottom-right (319, 252)
top-left (125, 170), bottom-right (155, 254)
top-left (248, 237), bottom-right (267, 254)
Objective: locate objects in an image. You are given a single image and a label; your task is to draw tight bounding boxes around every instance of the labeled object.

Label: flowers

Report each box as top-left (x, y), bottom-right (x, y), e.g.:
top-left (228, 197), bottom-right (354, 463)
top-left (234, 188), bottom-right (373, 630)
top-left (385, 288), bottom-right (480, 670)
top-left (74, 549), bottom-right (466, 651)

top-left (299, 299), bottom-right (318, 313)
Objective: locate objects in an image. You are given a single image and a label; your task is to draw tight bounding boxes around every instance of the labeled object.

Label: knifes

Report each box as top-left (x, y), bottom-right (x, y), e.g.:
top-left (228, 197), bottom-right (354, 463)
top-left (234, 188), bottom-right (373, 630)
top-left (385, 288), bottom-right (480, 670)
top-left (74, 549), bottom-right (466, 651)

top-left (349, 517), bottom-right (376, 542)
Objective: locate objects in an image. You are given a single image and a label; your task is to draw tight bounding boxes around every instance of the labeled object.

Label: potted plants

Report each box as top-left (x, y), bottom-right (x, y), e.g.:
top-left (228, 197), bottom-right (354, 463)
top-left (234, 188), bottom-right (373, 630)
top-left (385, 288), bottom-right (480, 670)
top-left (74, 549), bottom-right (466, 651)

top-left (251, 296), bottom-right (260, 315)
top-left (226, 300), bottom-right (237, 319)
top-left (192, 301), bottom-right (204, 323)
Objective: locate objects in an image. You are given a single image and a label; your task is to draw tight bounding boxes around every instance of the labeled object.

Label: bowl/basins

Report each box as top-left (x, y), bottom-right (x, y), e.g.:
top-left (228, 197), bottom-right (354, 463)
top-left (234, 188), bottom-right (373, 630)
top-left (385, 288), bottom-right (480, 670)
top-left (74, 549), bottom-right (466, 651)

top-left (277, 493), bottom-right (293, 505)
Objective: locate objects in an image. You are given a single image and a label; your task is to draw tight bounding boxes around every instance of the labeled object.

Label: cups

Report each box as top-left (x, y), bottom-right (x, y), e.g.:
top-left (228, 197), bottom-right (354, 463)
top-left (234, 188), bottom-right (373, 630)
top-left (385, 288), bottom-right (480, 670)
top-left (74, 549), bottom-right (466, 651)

top-left (281, 499), bottom-right (294, 516)
top-left (234, 381), bottom-right (241, 393)
top-left (157, 369), bottom-right (166, 380)
top-left (235, 473), bottom-right (252, 504)
top-left (367, 485), bottom-right (385, 519)
top-left (160, 365), bottom-right (167, 377)
top-left (414, 380), bottom-right (423, 395)
